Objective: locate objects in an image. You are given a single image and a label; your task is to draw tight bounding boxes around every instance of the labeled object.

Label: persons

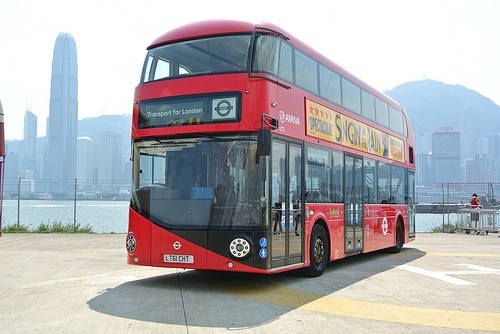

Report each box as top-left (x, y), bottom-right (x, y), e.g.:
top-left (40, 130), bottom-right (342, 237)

top-left (274, 195), bottom-right (283, 234)
top-left (293, 199), bottom-right (302, 236)
top-left (469, 194), bottom-right (480, 233)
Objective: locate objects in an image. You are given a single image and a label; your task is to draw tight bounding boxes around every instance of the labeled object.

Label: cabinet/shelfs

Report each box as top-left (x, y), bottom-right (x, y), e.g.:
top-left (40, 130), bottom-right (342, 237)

top-left (458, 205), bottom-right (500, 236)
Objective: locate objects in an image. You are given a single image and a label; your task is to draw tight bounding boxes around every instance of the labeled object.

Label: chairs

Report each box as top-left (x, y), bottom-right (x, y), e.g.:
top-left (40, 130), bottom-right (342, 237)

top-left (173, 165), bottom-right (197, 199)
top-left (305, 192), bottom-right (319, 202)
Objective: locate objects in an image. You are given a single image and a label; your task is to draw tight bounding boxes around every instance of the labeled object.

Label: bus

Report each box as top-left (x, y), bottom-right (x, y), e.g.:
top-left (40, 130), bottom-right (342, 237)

top-left (126, 18), bottom-right (418, 277)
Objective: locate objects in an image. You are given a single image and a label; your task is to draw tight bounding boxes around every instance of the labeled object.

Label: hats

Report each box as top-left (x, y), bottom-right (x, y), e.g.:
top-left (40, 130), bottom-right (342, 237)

top-left (472, 193), bottom-right (476, 196)
